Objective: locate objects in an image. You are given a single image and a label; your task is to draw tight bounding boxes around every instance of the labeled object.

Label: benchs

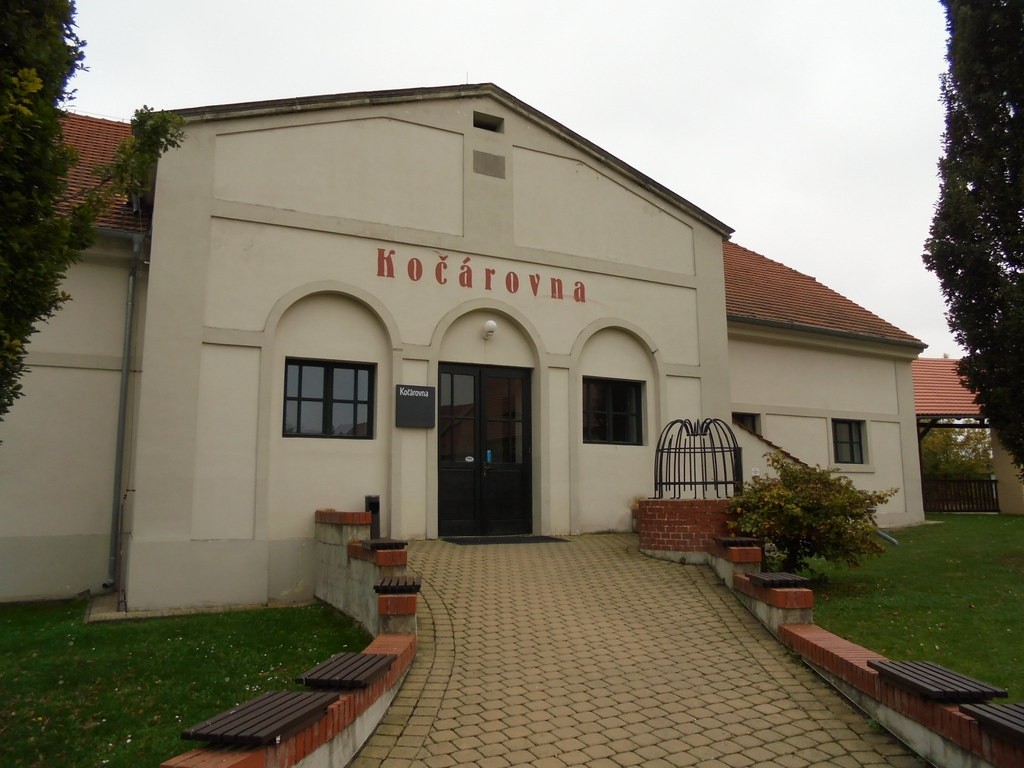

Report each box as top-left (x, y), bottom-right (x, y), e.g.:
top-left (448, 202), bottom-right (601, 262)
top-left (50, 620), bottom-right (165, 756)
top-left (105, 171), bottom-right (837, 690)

top-left (372, 574), bottom-right (422, 594)
top-left (744, 571), bottom-right (807, 587)
top-left (957, 701), bottom-right (1024, 744)
top-left (179, 688), bottom-right (340, 748)
top-left (867, 658), bottom-right (1009, 704)
top-left (711, 535), bottom-right (761, 548)
top-left (294, 651), bottom-right (398, 692)
top-left (368, 537), bottom-right (409, 551)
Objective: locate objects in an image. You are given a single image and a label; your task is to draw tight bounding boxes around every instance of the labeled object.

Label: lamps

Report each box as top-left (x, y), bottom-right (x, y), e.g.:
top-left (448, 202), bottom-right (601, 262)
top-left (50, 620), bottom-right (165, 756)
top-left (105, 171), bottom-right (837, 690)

top-left (482, 320), bottom-right (496, 340)
